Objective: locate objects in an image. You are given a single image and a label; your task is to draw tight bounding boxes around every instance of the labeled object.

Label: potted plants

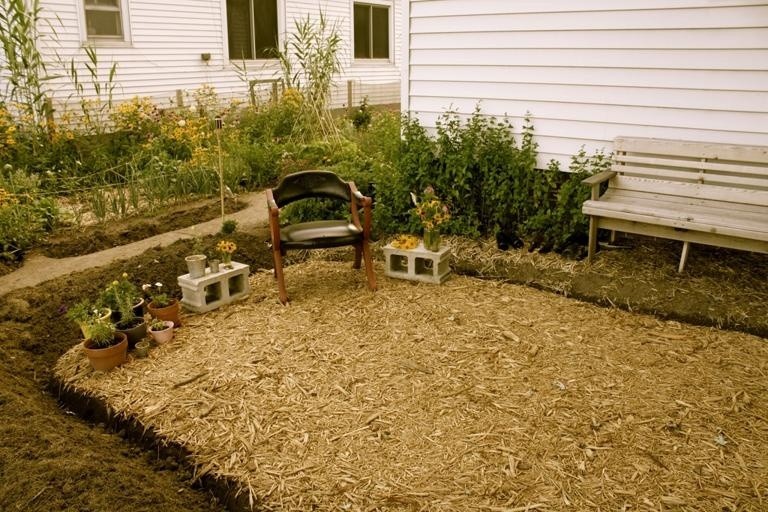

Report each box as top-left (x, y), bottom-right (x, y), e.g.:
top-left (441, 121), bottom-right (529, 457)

top-left (96, 281), bottom-right (146, 323)
top-left (134, 338), bottom-right (150, 358)
top-left (185, 216), bottom-right (208, 278)
top-left (67, 297), bottom-right (112, 339)
top-left (149, 316), bottom-right (175, 343)
top-left (207, 250), bottom-right (220, 273)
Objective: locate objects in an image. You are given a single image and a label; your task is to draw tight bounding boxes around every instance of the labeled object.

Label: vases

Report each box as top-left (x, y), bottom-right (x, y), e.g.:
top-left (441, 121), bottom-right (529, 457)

top-left (221, 253), bottom-right (233, 270)
top-left (147, 299), bottom-right (180, 328)
top-left (81, 331), bottom-right (129, 371)
top-left (114, 316), bottom-right (147, 349)
top-left (423, 227), bottom-right (441, 251)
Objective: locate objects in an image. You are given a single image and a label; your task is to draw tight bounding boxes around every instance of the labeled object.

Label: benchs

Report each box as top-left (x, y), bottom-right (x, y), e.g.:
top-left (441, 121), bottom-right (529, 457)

top-left (581, 136), bottom-right (768, 275)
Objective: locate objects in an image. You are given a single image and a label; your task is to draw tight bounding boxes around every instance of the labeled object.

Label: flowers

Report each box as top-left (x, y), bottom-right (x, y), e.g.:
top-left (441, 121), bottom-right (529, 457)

top-left (84, 316), bottom-right (118, 348)
top-left (141, 282), bottom-right (172, 306)
top-left (216, 239), bottom-right (237, 254)
top-left (407, 184), bottom-right (453, 230)
top-left (391, 233), bottom-right (421, 250)
top-left (111, 272), bottom-right (138, 327)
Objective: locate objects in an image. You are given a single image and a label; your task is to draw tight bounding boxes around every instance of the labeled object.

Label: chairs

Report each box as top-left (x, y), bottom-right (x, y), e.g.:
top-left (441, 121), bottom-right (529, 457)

top-left (265, 170), bottom-right (378, 305)
